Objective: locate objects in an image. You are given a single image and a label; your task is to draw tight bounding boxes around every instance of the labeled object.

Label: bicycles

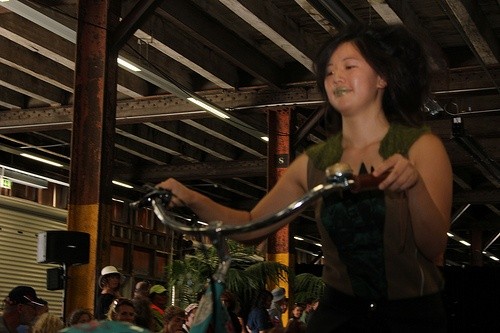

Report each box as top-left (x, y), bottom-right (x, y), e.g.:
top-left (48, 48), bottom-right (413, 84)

top-left (129, 162), bottom-right (394, 333)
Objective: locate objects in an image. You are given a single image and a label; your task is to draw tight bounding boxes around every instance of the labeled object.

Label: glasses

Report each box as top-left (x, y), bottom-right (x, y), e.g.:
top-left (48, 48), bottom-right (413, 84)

top-left (112, 299), bottom-right (132, 305)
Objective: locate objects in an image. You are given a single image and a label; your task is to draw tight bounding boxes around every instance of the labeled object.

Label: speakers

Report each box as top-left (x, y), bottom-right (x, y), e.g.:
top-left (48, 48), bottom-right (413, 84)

top-left (37, 231), bottom-right (90, 265)
top-left (47, 267), bottom-right (63, 290)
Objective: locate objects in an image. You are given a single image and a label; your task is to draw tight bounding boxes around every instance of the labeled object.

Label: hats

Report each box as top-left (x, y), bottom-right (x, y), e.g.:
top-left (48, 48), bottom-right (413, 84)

top-left (273, 287), bottom-right (284, 302)
top-left (163, 305), bottom-right (184, 319)
top-left (97, 266), bottom-right (123, 289)
top-left (148, 284), bottom-right (169, 294)
top-left (184, 303), bottom-right (199, 315)
top-left (8, 287), bottom-right (47, 306)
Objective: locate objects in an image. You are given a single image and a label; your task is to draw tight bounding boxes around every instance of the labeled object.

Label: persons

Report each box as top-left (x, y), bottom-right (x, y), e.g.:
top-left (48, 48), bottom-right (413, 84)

top-left (0, 266), bottom-right (320, 333)
top-left (153, 23), bottom-right (453, 333)
